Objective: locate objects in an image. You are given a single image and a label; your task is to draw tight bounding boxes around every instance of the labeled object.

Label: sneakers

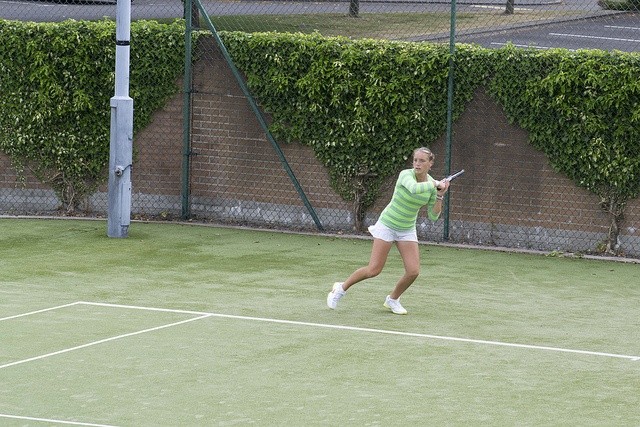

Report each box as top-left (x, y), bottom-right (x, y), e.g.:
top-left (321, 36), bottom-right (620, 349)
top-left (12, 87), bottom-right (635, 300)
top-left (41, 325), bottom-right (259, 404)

top-left (383, 295), bottom-right (407, 315)
top-left (327, 282), bottom-right (346, 310)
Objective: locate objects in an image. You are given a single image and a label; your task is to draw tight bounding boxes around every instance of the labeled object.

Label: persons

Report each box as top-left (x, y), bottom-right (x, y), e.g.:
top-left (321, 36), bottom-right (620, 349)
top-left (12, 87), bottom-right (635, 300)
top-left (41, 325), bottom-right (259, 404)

top-left (326, 147), bottom-right (450, 315)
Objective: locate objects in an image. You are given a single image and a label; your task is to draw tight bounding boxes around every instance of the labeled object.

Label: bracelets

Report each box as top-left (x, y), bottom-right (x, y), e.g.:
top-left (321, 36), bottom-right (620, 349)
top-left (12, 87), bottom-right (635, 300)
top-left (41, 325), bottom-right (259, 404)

top-left (437, 196), bottom-right (444, 201)
